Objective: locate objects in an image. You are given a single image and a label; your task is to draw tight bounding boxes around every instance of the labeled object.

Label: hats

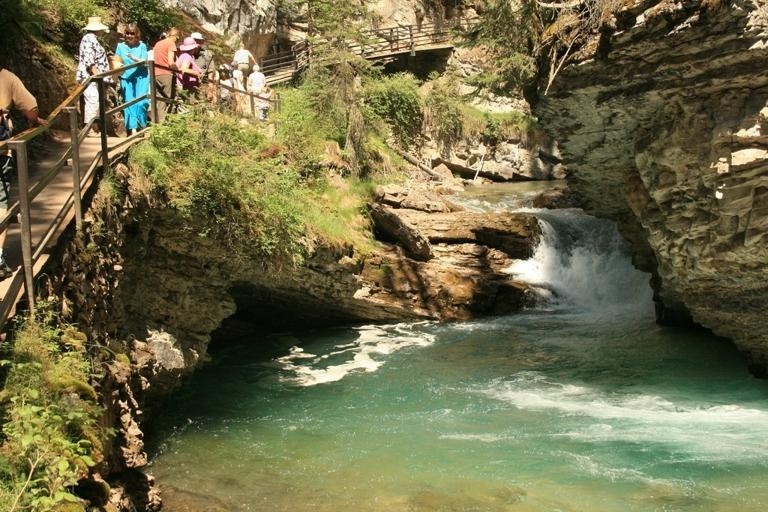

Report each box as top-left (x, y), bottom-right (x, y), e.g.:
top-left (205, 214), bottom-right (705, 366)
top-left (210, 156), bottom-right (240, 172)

top-left (191, 33), bottom-right (204, 40)
top-left (179, 37), bottom-right (201, 51)
top-left (218, 64), bottom-right (233, 70)
top-left (107, 55), bottom-right (125, 83)
top-left (81, 16), bottom-right (109, 32)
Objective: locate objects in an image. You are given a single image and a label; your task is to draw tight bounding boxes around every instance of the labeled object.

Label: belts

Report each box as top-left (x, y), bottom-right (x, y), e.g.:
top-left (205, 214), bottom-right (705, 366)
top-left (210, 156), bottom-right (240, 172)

top-left (239, 63), bottom-right (248, 64)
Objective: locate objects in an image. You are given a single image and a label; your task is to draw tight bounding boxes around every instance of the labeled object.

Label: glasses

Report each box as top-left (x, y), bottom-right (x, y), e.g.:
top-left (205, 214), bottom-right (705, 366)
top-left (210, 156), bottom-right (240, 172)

top-left (126, 31), bottom-right (136, 35)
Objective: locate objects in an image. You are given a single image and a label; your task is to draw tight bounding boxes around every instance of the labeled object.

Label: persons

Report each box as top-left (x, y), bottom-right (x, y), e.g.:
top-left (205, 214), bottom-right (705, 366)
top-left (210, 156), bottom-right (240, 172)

top-left (232, 43), bottom-right (257, 75)
top-left (0, 62), bottom-right (50, 281)
top-left (212, 62), bottom-right (274, 122)
top-left (148, 26), bottom-right (217, 124)
top-left (109, 22), bottom-right (151, 138)
top-left (74, 16), bottom-right (116, 139)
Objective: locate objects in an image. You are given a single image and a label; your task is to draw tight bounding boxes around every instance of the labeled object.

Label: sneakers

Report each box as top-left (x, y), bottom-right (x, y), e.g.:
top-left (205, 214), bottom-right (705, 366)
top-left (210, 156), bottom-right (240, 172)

top-left (87, 128), bottom-right (109, 137)
top-left (0, 257), bottom-right (13, 279)
